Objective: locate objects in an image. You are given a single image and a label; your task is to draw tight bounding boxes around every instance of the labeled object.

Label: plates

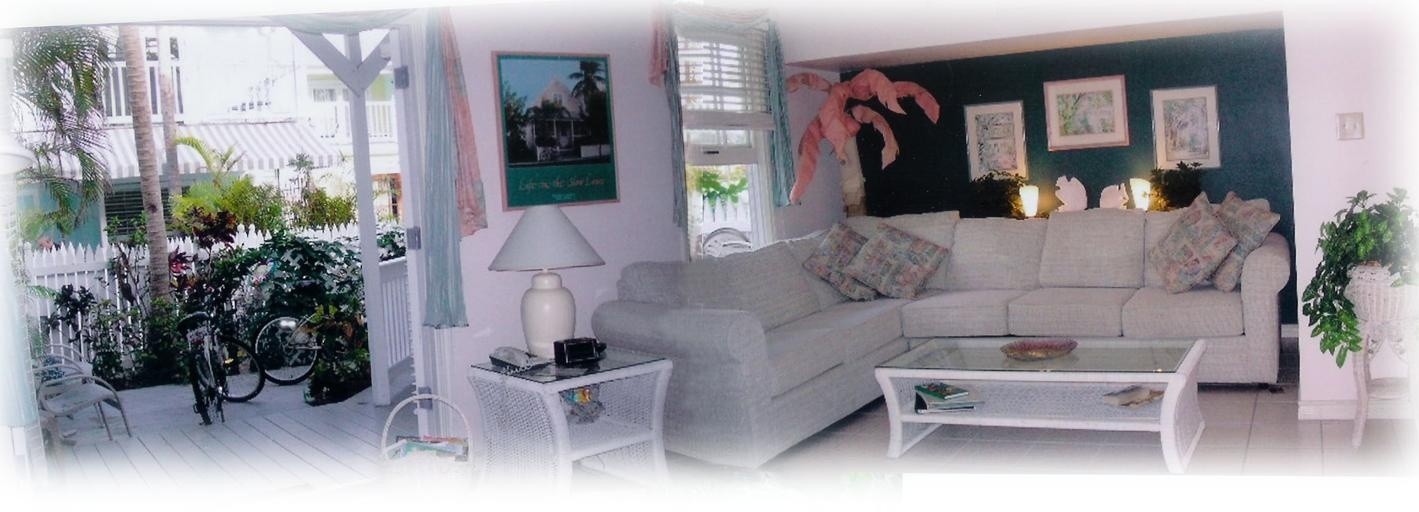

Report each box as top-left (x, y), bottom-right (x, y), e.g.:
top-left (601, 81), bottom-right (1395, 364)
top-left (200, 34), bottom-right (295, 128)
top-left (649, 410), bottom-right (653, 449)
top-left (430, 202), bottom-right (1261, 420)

top-left (1001, 339), bottom-right (1077, 360)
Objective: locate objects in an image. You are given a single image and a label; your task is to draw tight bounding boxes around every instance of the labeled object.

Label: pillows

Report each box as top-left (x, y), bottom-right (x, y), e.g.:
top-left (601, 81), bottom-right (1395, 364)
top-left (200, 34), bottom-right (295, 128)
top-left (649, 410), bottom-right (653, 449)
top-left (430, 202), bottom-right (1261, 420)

top-left (1210, 191), bottom-right (1282, 292)
top-left (802, 223), bottom-right (880, 301)
top-left (1148, 190), bottom-right (1239, 292)
top-left (846, 223), bottom-right (949, 300)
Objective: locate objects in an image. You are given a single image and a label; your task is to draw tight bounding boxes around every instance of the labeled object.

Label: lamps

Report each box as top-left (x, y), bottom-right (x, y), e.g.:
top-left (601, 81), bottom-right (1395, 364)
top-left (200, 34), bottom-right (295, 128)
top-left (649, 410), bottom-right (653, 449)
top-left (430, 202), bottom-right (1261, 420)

top-left (488, 204), bottom-right (605, 363)
top-left (1019, 185), bottom-right (1039, 220)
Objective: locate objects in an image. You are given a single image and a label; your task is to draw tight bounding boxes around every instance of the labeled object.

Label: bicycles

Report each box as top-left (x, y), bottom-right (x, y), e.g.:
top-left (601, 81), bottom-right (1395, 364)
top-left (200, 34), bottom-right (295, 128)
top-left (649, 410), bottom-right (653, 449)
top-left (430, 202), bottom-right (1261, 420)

top-left (180, 289), bottom-right (266, 425)
top-left (253, 282), bottom-right (368, 384)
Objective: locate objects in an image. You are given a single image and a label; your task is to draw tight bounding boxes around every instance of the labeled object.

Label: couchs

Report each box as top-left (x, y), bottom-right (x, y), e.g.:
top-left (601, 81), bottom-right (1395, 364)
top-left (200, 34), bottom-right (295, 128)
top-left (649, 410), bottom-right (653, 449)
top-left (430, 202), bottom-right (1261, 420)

top-left (902, 216), bottom-right (1290, 391)
top-left (590, 230), bottom-right (912, 480)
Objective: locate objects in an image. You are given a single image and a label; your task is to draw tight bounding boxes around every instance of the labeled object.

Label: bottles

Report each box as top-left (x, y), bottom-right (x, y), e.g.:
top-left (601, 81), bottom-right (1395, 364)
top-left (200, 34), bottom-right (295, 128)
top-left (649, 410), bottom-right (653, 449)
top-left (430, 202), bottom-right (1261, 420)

top-left (553, 338), bottom-right (606, 364)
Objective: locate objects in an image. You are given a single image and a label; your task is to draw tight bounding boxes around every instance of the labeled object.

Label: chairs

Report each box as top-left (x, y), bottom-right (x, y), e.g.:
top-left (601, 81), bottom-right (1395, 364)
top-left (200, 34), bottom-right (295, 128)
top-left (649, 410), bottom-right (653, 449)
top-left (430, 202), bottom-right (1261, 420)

top-left (11, 365), bottom-right (133, 469)
top-left (26, 343), bottom-right (105, 442)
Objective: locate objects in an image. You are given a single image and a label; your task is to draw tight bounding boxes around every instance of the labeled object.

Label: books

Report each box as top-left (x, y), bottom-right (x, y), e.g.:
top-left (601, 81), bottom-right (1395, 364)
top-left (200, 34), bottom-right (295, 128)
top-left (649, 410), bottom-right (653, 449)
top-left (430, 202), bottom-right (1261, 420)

top-left (1119, 389), bottom-right (1164, 410)
top-left (913, 380), bottom-right (985, 415)
top-left (1103, 385), bottom-right (1151, 407)
top-left (393, 434), bottom-right (469, 462)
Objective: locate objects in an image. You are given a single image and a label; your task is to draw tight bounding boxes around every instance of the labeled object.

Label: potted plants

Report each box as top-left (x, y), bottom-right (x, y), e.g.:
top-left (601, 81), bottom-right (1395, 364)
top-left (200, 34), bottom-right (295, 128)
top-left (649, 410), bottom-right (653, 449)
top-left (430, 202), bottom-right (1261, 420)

top-left (1302, 186), bottom-right (1419, 371)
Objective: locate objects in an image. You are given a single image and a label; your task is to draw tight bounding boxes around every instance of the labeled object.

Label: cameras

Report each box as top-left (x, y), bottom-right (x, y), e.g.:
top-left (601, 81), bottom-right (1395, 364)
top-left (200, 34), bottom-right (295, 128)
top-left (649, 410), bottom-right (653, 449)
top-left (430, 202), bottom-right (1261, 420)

top-left (553, 337), bottom-right (613, 366)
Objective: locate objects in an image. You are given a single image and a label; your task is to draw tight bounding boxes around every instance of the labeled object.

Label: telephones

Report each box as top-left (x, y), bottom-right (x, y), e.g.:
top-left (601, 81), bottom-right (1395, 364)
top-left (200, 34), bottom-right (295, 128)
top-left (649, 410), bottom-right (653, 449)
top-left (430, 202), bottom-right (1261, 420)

top-left (489, 347), bottom-right (549, 372)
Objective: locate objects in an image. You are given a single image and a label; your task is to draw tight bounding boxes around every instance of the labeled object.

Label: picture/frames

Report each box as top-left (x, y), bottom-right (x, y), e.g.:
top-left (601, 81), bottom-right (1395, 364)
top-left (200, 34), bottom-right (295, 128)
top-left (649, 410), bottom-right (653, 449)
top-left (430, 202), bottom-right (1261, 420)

top-left (964, 100), bottom-right (1030, 184)
top-left (1150, 85), bottom-right (1222, 174)
top-left (1043, 74), bottom-right (1131, 153)
top-left (491, 49), bottom-right (622, 212)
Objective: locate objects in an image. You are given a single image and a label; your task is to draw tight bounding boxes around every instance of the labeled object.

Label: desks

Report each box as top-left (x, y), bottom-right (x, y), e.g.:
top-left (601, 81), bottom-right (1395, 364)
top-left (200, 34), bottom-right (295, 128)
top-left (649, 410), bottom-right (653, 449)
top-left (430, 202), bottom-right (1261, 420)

top-left (468, 345), bottom-right (673, 494)
